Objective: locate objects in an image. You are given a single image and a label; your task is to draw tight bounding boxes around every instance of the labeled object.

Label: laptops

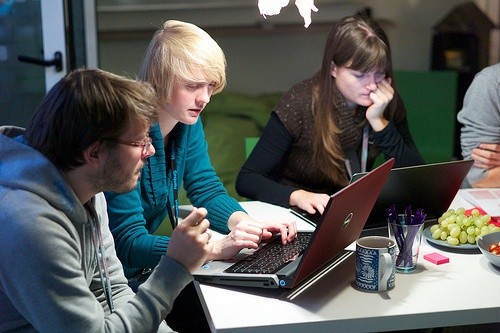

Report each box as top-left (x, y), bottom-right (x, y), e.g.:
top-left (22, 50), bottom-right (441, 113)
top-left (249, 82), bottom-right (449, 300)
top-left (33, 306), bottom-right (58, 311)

top-left (290, 157), bottom-right (474, 232)
top-left (189, 158), bottom-right (395, 289)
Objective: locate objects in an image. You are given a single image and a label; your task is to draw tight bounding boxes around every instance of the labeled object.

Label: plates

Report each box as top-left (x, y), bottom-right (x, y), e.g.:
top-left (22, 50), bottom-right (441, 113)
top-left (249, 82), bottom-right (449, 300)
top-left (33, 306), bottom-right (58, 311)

top-left (423, 224), bottom-right (479, 249)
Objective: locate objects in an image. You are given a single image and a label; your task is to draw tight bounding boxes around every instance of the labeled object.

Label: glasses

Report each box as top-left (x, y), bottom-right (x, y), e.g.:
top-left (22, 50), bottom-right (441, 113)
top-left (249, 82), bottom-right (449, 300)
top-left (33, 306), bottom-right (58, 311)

top-left (100, 135), bottom-right (155, 151)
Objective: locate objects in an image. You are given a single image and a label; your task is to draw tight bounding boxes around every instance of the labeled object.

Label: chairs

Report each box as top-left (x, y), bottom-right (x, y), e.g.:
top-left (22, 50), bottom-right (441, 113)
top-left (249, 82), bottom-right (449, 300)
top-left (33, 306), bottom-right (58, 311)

top-left (371, 70), bottom-right (456, 170)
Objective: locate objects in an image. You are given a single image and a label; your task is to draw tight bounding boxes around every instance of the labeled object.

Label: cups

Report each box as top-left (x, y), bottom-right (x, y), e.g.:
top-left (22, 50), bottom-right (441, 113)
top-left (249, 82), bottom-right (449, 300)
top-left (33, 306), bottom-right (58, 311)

top-left (387, 214), bottom-right (424, 273)
top-left (355, 236), bottom-right (397, 292)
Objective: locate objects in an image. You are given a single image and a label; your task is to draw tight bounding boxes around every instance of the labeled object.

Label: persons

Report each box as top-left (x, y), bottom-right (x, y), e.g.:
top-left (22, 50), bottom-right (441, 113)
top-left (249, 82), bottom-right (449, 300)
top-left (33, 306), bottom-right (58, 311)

top-left (457, 64), bottom-right (500, 189)
top-left (235, 14), bottom-right (425, 216)
top-left (1, 69), bottom-right (213, 333)
top-left (103, 21), bottom-right (298, 333)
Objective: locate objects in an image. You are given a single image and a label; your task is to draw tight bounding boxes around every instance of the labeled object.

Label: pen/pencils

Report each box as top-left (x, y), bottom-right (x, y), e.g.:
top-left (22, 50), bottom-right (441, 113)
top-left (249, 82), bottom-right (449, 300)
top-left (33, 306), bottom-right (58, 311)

top-left (387, 204), bottom-right (428, 269)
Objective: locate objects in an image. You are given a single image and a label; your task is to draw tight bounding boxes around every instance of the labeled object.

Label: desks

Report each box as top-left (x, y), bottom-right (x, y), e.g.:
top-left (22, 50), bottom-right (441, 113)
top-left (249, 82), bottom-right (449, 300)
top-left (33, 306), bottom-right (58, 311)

top-left (172, 187), bottom-right (500, 333)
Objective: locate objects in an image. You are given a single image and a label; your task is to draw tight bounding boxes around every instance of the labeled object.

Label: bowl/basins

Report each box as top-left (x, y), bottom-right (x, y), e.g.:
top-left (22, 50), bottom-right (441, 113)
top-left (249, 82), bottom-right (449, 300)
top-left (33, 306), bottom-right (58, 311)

top-left (477, 231), bottom-right (500, 269)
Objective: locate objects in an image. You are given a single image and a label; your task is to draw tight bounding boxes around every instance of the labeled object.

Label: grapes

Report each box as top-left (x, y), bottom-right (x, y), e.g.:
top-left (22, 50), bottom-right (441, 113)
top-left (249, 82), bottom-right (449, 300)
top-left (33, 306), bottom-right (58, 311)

top-left (430, 207), bottom-right (500, 246)
